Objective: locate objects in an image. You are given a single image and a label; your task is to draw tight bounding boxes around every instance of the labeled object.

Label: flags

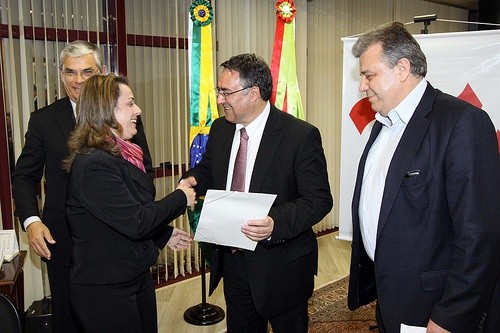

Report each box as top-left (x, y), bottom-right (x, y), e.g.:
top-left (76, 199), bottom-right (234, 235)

top-left (270, 0), bottom-right (305, 122)
top-left (187, 0), bottom-right (222, 237)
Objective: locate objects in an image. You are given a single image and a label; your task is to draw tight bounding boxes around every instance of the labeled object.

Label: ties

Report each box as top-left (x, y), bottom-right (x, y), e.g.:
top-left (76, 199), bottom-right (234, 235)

top-left (231, 127), bottom-right (249, 192)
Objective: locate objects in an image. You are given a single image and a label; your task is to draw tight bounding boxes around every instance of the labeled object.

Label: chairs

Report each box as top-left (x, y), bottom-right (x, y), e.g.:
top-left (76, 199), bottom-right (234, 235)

top-left (0, 293), bottom-right (22, 333)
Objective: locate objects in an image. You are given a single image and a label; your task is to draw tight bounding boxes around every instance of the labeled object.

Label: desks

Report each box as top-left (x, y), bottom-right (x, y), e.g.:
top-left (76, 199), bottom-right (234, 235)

top-left (0, 250), bottom-right (28, 319)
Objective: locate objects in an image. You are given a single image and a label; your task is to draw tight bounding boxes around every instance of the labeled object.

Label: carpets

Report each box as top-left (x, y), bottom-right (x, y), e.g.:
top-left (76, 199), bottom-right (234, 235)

top-left (224, 276), bottom-right (379, 333)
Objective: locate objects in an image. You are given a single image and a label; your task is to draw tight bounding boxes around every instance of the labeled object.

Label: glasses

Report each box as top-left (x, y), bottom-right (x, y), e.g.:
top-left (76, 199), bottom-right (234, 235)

top-left (215, 84), bottom-right (255, 99)
top-left (62, 69), bottom-right (99, 80)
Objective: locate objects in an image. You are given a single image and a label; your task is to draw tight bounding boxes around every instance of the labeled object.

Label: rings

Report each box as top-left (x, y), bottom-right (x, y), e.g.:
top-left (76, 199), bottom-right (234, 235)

top-left (175, 244), bottom-right (178, 248)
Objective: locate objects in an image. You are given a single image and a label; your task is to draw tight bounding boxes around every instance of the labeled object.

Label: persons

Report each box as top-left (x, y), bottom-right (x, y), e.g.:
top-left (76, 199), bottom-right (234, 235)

top-left (13, 41), bottom-right (154, 333)
top-left (178, 53), bottom-right (333, 333)
top-left (6, 73), bottom-right (59, 202)
top-left (348, 21), bottom-right (500, 333)
top-left (62, 74), bottom-right (197, 333)
